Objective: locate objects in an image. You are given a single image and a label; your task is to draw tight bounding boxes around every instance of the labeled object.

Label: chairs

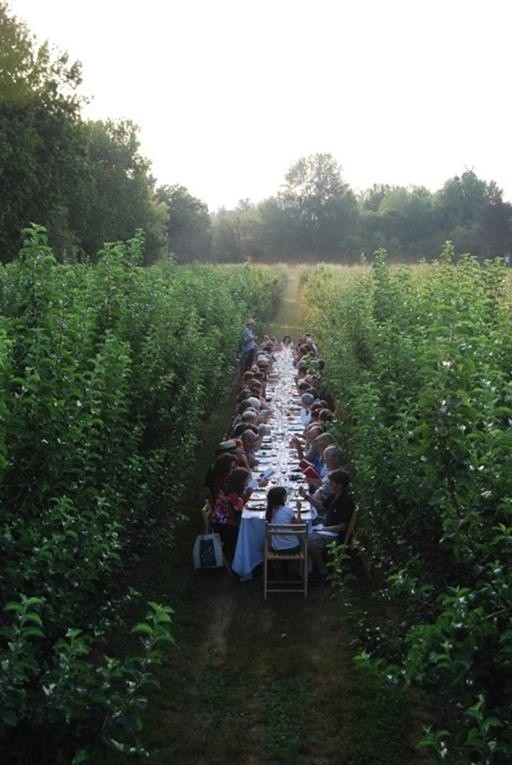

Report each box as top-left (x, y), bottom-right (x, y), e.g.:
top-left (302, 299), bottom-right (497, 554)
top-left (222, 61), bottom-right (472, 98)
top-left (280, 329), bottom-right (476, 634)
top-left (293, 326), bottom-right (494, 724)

top-left (202, 340), bottom-right (361, 606)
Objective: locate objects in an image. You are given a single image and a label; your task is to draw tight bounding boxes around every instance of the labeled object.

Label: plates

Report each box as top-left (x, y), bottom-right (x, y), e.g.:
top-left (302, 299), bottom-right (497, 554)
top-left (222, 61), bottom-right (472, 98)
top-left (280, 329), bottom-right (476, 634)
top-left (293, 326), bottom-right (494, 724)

top-left (244, 450), bottom-right (312, 512)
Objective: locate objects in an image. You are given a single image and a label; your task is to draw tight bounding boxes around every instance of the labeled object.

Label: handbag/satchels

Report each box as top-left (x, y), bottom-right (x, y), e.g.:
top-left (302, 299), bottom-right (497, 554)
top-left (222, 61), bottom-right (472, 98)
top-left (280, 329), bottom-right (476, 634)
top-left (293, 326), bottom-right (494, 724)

top-left (192, 534), bottom-right (225, 570)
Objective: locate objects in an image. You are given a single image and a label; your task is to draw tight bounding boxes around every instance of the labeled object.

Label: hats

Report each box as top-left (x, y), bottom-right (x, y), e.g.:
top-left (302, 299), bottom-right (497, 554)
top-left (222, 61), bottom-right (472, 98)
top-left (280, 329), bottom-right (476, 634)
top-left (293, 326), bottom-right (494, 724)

top-left (218, 319), bottom-right (344, 450)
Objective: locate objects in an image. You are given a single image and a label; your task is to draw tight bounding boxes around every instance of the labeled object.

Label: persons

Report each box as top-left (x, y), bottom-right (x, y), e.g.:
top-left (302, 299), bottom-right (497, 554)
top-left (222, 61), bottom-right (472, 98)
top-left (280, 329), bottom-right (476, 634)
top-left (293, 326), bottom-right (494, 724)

top-left (209, 318), bottom-right (354, 582)
